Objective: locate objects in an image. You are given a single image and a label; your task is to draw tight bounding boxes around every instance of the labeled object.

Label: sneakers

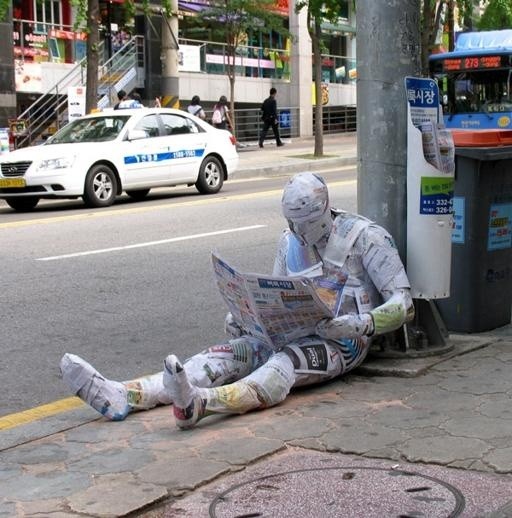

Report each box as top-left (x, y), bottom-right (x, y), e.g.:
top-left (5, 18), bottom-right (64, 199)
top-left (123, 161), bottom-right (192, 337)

top-left (259, 139), bottom-right (264, 147)
top-left (277, 141), bottom-right (285, 146)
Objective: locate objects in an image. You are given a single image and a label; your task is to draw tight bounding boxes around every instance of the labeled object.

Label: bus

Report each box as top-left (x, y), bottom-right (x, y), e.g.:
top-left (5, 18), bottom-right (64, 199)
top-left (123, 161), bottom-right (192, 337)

top-left (424, 27), bottom-right (512, 128)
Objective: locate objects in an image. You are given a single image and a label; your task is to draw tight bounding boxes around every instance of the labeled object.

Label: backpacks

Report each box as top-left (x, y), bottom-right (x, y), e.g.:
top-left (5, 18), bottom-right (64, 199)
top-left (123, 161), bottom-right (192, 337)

top-left (211, 105), bottom-right (223, 124)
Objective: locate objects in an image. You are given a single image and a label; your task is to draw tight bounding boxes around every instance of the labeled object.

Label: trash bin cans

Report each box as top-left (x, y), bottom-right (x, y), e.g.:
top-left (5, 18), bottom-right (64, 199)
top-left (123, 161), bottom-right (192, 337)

top-left (432, 128), bottom-right (512, 334)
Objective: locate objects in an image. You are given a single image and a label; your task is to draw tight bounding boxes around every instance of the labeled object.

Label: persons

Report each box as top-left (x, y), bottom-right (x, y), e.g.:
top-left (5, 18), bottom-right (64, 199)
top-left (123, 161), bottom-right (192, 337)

top-left (58, 171), bottom-right (415, 431)
top-left (114, 89), bottom-right (235, 135)
top-left (259, 87), bottom-right (285, 148)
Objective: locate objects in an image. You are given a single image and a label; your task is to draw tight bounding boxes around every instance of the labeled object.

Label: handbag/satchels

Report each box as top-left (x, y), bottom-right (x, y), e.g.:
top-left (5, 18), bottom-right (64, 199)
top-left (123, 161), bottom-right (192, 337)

top-left (261, 110), bottom-right (277, 124)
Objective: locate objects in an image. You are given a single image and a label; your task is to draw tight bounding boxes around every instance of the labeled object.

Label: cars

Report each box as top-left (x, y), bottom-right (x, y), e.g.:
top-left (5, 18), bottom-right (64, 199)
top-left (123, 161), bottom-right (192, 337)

top-left (0, 105), bottom-right (243, 207)
